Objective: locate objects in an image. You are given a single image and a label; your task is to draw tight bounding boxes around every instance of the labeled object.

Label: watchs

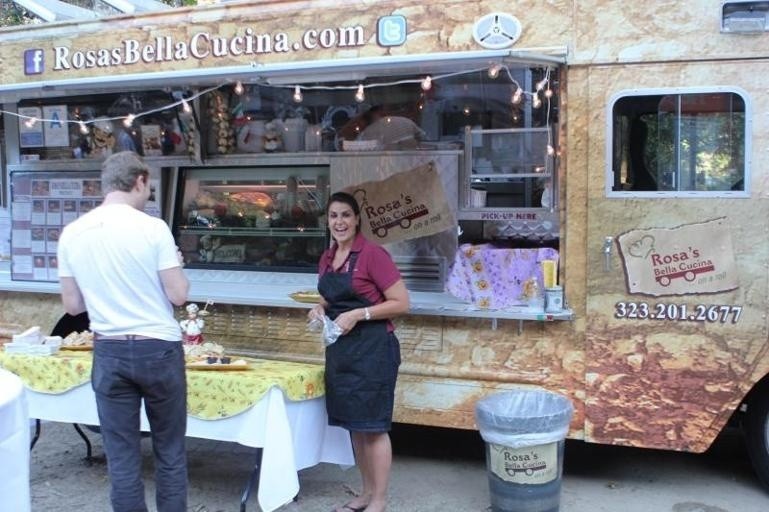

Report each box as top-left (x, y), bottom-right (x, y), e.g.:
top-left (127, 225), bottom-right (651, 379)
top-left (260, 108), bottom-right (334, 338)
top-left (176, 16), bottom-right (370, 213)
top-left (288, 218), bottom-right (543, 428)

top-left (364, 307), bottom-right (371, 321)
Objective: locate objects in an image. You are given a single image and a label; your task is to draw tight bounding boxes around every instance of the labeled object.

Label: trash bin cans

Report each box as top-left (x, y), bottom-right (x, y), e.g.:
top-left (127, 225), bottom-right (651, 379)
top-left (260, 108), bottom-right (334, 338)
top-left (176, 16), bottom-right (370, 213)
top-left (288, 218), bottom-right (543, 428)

top-left (475, 388), bottom-right (574, 512)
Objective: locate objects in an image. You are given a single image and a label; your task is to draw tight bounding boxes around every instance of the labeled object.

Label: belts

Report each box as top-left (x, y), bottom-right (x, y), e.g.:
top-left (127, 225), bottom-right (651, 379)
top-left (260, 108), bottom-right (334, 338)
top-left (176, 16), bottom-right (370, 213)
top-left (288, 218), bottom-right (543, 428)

top-left (94, 333), bottom-right (154, 341)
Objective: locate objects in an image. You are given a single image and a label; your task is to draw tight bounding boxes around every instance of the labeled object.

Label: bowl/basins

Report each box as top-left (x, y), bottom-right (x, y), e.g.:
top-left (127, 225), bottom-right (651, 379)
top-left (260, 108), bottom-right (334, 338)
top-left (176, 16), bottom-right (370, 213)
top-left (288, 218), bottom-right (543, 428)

top-left (238, 118), bottom-right (327, 152)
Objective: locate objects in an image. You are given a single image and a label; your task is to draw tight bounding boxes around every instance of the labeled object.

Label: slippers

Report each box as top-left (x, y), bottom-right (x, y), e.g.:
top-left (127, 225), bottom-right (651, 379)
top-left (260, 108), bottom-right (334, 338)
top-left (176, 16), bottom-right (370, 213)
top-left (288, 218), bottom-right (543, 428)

top-left (330, 502), bottom-right (367, 512)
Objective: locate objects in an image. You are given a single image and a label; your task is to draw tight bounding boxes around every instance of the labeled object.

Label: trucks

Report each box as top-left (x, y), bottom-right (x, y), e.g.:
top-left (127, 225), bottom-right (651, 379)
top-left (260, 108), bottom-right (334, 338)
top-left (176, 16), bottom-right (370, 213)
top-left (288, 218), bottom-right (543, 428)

top-left (2, 0), bottom-right (767, 498)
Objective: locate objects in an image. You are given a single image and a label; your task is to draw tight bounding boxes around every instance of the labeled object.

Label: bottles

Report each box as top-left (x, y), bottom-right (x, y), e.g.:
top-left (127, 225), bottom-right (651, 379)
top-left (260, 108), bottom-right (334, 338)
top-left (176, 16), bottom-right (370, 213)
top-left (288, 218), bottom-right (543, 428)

top-left (543, 286), bottom-right (563, 313)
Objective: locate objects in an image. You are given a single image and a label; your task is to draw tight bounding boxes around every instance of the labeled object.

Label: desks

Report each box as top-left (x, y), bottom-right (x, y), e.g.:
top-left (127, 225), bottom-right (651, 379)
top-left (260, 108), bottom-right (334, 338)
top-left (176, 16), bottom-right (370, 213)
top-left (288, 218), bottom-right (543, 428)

top-left (0, 346), bottom-right (359, 511)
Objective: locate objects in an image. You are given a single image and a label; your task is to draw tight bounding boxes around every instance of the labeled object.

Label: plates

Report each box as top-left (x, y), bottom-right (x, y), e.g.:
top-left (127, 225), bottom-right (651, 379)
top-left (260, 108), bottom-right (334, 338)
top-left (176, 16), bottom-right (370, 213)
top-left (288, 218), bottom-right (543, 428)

top-left (288, 290), bottom-right (322, 304)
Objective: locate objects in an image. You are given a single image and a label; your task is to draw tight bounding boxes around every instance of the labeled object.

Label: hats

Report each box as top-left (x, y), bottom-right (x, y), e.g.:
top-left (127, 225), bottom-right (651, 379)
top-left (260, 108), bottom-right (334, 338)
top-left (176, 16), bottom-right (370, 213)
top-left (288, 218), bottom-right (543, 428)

top-left (94, 115), bottom-right (113, 133)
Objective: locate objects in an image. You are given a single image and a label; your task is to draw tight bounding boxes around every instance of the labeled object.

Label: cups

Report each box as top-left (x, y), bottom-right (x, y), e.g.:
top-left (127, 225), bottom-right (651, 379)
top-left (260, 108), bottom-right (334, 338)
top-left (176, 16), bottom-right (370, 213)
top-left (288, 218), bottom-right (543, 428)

top-left (542, 259), bottom-right (556, 289)
top-left (285, 175), bottom-right (299, 215)
top-left (314, 173), bottom-right (327, 210)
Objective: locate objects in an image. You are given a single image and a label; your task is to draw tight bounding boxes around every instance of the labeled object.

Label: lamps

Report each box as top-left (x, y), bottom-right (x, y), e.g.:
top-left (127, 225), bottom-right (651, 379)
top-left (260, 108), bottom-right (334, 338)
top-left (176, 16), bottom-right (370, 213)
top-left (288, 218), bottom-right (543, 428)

top-left (0, 63), bottom-right (557, 173)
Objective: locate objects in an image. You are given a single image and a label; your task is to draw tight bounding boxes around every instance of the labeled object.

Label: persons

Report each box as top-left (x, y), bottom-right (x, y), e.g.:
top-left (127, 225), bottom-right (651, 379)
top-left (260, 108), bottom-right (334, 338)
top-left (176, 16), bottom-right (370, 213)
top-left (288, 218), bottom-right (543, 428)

top-left (179, 303), bottom-right (208, 344)
top-left (59, 151), bottom-right (192, 511)
top-left (308, 192), bottom-right (408, 511)
